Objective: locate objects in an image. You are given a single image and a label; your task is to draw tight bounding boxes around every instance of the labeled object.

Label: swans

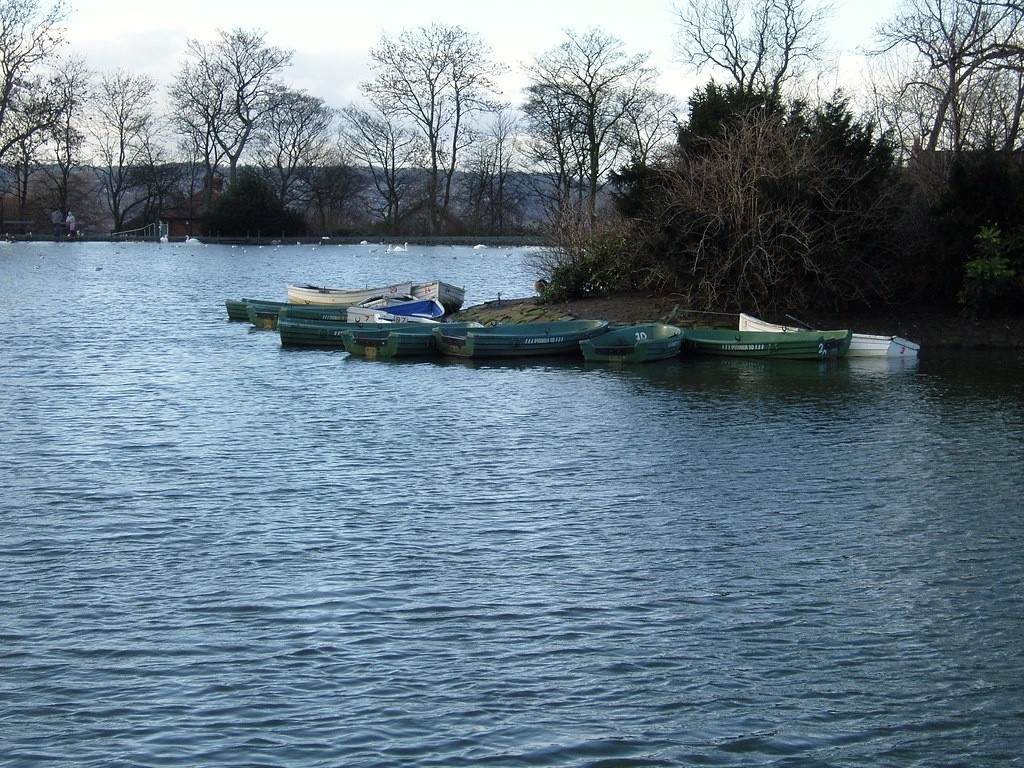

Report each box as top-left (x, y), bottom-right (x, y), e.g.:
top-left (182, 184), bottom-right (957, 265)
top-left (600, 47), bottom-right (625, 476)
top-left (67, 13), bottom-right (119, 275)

top-left (387, 244), bottom-right (393, 252)
top-left (393, 241), bottom-right (408, 251)
top-left (360, 241), bottom-right (367, 245)
top-left (473, 244), bottom-right (488, 250)
top-left (184, 235), bottom-right (200, 244)
top-left (160, 234), bottom-right (168, 243)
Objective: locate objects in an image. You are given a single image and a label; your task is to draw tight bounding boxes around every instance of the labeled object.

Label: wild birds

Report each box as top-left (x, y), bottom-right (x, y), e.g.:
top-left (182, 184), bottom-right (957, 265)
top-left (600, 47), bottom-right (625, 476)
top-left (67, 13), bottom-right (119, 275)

top-left (0, 231), bottom-right (567, 273)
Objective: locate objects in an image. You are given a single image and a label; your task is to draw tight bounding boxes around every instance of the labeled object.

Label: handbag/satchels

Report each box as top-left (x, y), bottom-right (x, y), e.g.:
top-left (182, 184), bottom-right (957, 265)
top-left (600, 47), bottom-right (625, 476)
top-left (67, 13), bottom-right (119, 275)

top-left (70, 222), bottom-right (75, 230)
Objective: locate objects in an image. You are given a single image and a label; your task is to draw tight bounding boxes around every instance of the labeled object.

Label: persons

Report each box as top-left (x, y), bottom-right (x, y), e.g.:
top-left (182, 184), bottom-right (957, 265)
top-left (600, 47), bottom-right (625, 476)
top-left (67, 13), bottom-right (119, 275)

top-left (66, 212), bottom-right (75, 237)
top-left (52, 208), bottom-right (62, 237)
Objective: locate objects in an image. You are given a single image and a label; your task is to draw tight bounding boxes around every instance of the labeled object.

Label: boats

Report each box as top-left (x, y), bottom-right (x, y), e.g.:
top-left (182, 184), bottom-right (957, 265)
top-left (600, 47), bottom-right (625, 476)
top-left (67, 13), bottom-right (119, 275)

top-left (678, 329), bottom-right (853, 362)
top-left (579, 323), bottom-right (686, 365)
top-left (738, 311), bottom-right (921, 358)
top-left (431, 318), bottom-right (609, 359)
top-left (224, 278), bottom-right (467, 346)
top-left (340, 320), bottom-right (484, 359)
